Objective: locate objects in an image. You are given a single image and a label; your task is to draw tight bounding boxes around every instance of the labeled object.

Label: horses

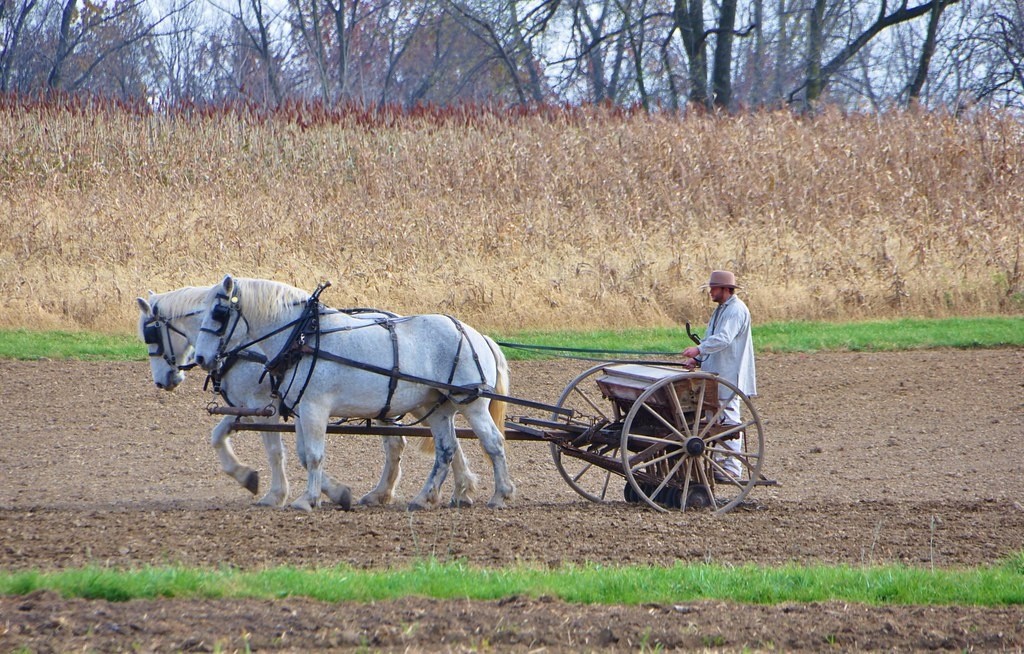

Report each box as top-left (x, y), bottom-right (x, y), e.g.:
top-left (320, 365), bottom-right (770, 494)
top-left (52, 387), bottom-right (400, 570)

top-left (135, 274), bottom-right (515, 512)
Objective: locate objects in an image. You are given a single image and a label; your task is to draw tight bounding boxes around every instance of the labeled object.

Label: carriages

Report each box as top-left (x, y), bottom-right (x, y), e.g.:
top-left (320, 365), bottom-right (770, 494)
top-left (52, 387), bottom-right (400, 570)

top-left (136, 275), bottom-right (765, 518)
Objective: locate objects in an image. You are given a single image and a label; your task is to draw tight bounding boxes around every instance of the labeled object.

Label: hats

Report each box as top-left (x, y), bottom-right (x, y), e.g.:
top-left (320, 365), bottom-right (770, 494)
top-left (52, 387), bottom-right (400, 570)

top-left (698, 270), bottom-right (744, 292)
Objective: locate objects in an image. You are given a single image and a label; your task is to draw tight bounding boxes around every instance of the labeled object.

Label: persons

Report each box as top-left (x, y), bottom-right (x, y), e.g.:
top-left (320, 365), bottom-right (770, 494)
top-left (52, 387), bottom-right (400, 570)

top-left (680, 271), bottom-right (760, 483)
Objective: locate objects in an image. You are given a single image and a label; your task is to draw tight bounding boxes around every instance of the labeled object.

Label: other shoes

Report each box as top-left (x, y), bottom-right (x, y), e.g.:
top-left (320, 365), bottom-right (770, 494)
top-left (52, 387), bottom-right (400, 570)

top-left (712, 469), bottom-right (739, 480)
